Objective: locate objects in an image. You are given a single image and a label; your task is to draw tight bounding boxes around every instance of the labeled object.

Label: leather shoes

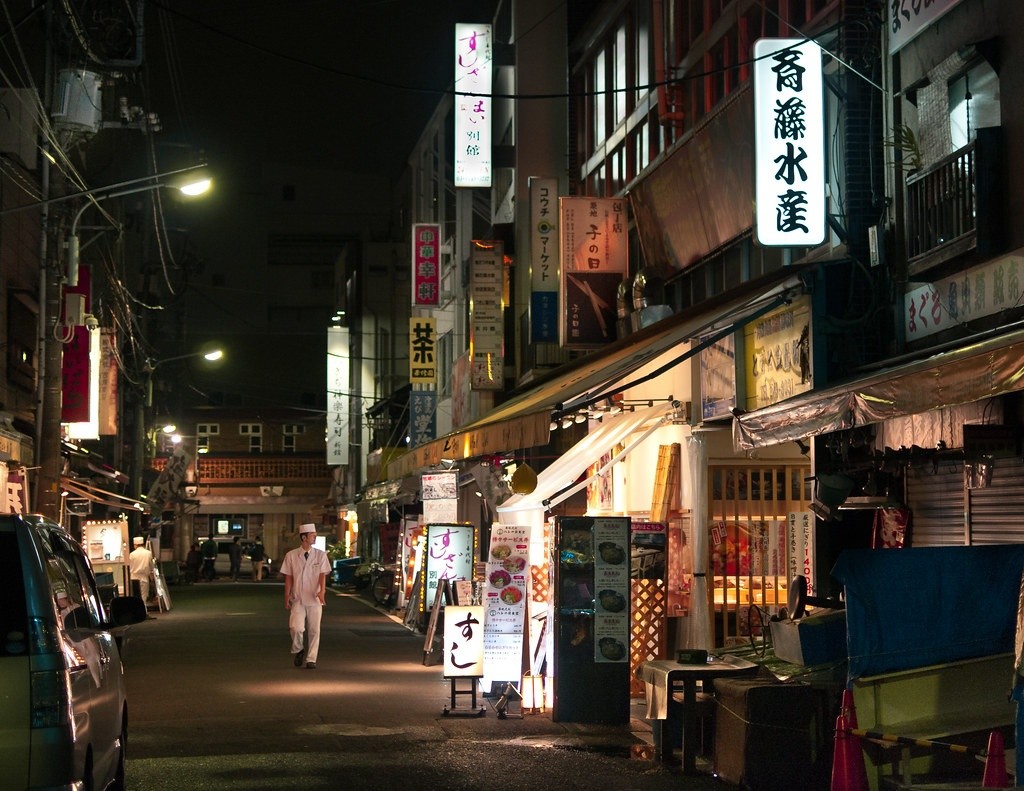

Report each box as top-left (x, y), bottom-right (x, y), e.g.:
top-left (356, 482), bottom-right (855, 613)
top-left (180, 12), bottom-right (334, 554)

top-left (306, 662), bottom-right (316, 668)
top-left (293, 650), bottom-right (305, 666)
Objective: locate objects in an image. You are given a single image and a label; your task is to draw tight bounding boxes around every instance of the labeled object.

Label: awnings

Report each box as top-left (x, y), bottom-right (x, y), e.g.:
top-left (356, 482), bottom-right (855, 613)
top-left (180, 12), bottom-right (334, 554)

top-left (732, 324), bottom-right (1023, 458)
top-left (385, 273), bottom-right (813, 491)
top-left (59, 480), bottom-right (148, 512)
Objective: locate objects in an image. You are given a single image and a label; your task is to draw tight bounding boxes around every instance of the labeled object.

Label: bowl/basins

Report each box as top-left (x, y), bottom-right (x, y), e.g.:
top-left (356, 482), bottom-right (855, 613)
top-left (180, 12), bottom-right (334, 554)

top-left (489, 546), bottom-right (527, 604)
top-left (598, 589), bottom-right (627, 613)
top-left (598, 637), bottom-right (627, 661)
top-left (597, 540), bottom-right (626, 565)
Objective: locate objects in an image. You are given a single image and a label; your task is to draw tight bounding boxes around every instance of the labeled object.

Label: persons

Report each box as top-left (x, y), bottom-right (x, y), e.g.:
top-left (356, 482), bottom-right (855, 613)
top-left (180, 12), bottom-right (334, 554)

top-left (228, 536), bottom-right (242, 580)
top-left (202, 533), bottom-right (218, 582)
top-left (668, 527), bottom-right (691, 574)
top-left (279, 523), bottom-right (332, 668)
top-left (250, 536), bottom-right (265, 580)
top-left (185, 542), bottom-right (203, 584)
top-left (128, 536), bottom-right (155, 620)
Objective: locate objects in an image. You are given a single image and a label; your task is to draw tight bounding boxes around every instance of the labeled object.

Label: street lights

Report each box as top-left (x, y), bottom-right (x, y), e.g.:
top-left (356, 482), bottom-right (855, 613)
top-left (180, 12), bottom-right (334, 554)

top-left (126, 344), bottom-right (226, 548)
top-left (30, 171), bottom-right (216, 524)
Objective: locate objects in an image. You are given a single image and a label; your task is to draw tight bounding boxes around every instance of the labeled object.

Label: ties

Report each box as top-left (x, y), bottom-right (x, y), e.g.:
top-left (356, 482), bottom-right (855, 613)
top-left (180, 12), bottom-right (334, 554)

top-left (303, 552), bottom-right (308, 559)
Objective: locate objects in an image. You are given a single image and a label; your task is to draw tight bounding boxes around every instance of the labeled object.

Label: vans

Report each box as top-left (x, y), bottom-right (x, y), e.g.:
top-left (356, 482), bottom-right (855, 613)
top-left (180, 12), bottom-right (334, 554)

top-left (0, 513), bottom-right (128, 791)
top-left (198, 537), bottom-right (272, 580)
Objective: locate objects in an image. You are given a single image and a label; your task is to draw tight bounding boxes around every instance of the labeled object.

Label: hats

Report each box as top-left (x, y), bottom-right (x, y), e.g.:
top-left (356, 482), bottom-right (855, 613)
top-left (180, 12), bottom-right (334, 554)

top-left (300, 523), bottom-right (315, 533)
top-left (134, 536), bottom-right (144, 544)
